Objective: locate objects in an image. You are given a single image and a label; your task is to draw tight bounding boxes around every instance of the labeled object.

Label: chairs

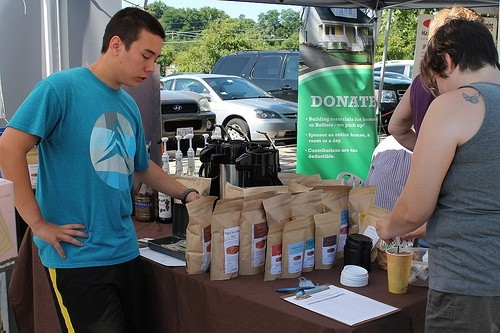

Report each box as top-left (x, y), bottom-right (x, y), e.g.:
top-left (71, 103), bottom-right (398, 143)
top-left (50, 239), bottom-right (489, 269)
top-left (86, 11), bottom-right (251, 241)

top-left (212, 85), bottom-right (225, 96)
top-left (188, 85), bottom-right (204, 93)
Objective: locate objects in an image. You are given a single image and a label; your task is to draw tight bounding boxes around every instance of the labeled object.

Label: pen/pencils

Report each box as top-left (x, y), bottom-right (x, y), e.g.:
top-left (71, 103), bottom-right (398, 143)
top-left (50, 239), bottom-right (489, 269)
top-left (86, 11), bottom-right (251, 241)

top-left (274, 286), bottom-right (316, 292)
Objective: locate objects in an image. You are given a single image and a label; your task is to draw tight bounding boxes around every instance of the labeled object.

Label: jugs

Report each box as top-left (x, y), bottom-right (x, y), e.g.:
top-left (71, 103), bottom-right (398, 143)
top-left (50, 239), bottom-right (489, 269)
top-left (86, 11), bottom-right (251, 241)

top-left (199, 138), bottom-right (280, 199)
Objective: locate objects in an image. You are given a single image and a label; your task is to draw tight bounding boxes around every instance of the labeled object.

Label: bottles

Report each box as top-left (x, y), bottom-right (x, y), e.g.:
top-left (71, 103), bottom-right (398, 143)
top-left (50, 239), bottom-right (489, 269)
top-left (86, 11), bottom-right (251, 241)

top-left (134, 142), bottom-right (158, 222)
top-left (344, 234), bottom-right (372, 273)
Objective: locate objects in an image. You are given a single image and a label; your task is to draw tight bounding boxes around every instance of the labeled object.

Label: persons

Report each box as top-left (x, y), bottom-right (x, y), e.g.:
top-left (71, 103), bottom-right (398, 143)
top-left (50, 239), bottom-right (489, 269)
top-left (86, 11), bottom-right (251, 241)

top-left (0, 7), bottom-right (206, 333)
top-left (366, 4), bottom-right (500, 333)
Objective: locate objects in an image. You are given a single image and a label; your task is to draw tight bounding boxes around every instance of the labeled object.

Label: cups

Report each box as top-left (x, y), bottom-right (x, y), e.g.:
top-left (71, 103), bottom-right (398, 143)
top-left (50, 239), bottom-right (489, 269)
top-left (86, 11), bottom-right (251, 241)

top-left (385, 247), bottom-right (414, 294)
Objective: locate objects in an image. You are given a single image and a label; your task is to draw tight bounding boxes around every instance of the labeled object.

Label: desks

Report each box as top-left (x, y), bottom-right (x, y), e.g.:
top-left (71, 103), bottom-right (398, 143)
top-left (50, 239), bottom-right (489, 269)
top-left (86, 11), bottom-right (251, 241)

top-left (9, 218), bottom-right (429, 333)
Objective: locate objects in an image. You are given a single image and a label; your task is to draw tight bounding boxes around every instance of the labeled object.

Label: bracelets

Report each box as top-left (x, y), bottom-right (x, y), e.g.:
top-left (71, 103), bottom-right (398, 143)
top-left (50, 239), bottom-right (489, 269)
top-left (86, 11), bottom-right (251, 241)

top-left (180, 188), bottom-right (199, 205)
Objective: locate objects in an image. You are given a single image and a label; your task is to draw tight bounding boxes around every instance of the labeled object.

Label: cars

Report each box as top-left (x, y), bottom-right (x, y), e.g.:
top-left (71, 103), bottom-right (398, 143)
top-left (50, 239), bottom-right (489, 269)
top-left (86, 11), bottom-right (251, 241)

top-left (160, 72), bottom-right (298, 155)
top-left (374, 60), bottom-right (414, 81)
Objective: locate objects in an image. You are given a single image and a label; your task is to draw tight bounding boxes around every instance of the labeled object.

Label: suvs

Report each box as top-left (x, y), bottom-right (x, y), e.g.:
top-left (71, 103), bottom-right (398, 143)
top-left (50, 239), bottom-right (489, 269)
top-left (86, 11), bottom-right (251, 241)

top-left (211, 50), bottom-right (413, 133)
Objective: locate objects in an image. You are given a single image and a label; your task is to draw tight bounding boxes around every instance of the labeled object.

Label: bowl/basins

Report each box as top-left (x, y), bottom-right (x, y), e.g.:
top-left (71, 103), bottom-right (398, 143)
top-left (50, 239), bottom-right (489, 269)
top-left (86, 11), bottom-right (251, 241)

top-left (410, 248), bottom-right (430, 288)
top-left (376, 244), bottom-right (387, 271)
